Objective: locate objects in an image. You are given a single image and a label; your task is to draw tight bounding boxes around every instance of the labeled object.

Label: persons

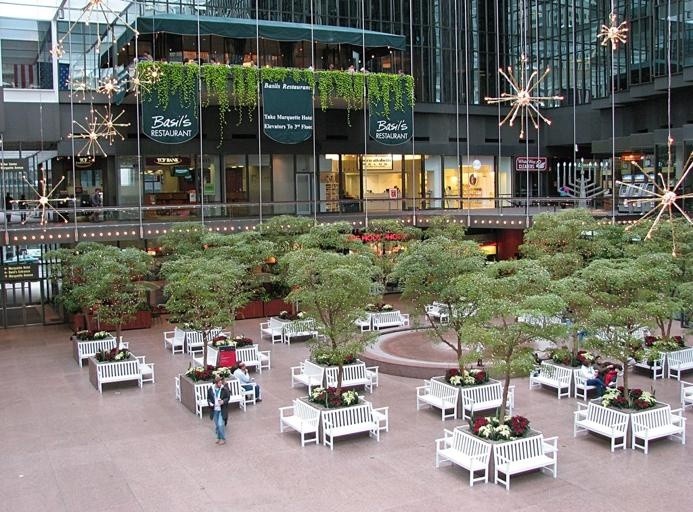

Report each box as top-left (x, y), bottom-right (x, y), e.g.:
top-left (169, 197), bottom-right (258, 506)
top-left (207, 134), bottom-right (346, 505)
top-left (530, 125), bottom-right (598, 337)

top-left (580, 359), bottom-right (612, 399)
top-left (87, 187), bottom-right (102, 223)
top-left (210, 57), bottom-right (216, 64)
top-left (347, 63), bottom-right (354, 72)
top-left (206, 376), bottom-right (231, 446)
top-left (17, 194), bottom-right (28, 222)
top-left (233, 362), bottom-right (263, 403)
top-left (142, 50), bottom-right (154, 61)
top-left (230, 360), bottom-right (242, 374)
top-left (591, 355), bottom-right (623, 382)
top-left (2, 192), bottom-right (14, 222)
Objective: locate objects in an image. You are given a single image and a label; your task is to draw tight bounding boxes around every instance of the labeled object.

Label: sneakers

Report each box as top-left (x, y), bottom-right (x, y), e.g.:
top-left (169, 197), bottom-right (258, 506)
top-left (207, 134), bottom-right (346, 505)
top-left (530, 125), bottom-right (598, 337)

top-left (215, 438), bottom-right (225, 446)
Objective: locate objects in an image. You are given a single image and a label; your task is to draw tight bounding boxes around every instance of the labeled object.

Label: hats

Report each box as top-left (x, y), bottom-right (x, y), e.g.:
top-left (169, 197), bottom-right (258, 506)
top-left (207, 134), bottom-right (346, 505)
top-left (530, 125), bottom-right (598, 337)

top-left (214, 376), bottom-right (224, 383)
